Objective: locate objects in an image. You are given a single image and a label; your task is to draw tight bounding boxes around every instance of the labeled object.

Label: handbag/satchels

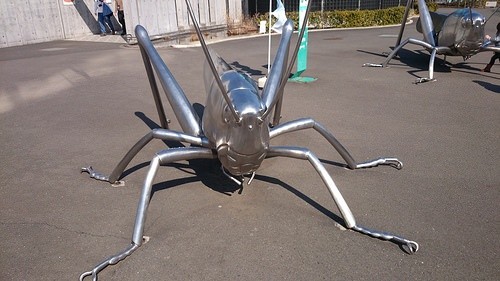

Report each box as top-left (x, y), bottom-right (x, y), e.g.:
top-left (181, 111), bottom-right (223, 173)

top-left (102, 2), bottom-right (113, 16)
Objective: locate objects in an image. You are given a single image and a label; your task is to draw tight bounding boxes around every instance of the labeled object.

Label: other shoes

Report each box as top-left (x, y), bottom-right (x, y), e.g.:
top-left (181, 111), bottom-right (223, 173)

top-left (110, 32), bottom-right (115, 35)
top-left (100, 33), bottom-right (106, 36)
top-left (121, 32), bottom-right (126, 35)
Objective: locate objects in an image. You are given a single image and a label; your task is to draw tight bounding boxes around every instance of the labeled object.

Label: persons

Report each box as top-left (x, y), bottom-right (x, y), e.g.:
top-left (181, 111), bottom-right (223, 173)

top-left (114, 0), bottom-right (126, 36)
top-left (480, 22), bottom-right (500, 72)
top-left (93, 0), bottom-right (115, 36)
